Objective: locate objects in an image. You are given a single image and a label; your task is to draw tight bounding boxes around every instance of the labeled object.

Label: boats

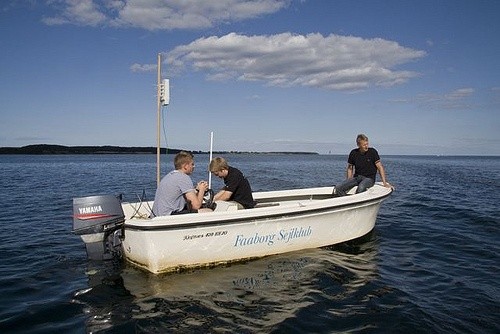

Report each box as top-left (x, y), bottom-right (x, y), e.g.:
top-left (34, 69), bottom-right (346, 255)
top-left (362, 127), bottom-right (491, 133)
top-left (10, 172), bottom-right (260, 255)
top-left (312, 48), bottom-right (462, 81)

top-left (73, 248), bottom-right (384, 334)
top-left (70, 52), bottom-right (394, 274)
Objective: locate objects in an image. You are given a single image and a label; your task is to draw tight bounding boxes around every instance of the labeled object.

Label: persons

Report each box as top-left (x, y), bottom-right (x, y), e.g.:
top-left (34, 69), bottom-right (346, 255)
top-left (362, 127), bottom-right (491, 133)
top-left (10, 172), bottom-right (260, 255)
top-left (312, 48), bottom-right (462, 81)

top-left (209, 157), bottom-right (254, 210)
top-left (333, 134), bottom-right (395, 197)
top-left (149, 152), bottom-right (214, 219)
